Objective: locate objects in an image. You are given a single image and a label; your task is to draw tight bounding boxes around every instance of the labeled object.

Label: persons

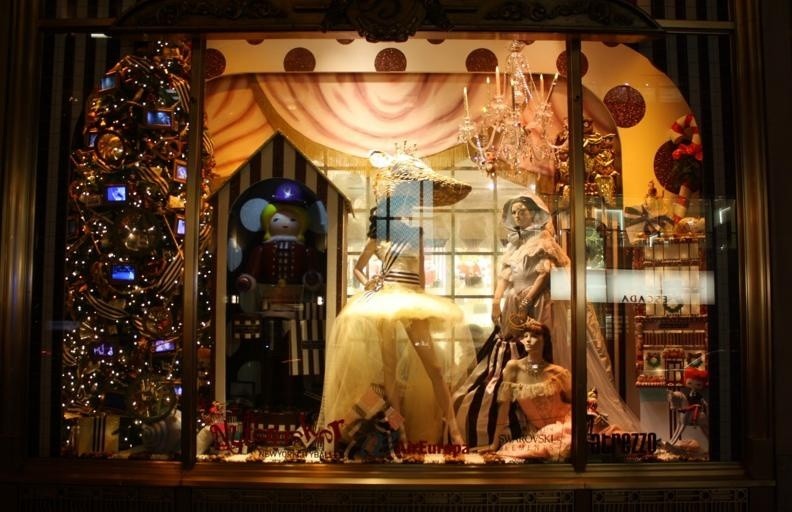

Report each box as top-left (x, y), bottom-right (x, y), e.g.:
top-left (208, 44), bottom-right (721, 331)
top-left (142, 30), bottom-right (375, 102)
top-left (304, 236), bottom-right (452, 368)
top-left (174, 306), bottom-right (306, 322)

top-left (243, 201), bottom-right (329, 285)
top-left (468, 318), bottom-right (655, 464)
top-left (442, 190), bottom-right (614, 447)
top-left (314, 195), bottom-right (477, 451)
